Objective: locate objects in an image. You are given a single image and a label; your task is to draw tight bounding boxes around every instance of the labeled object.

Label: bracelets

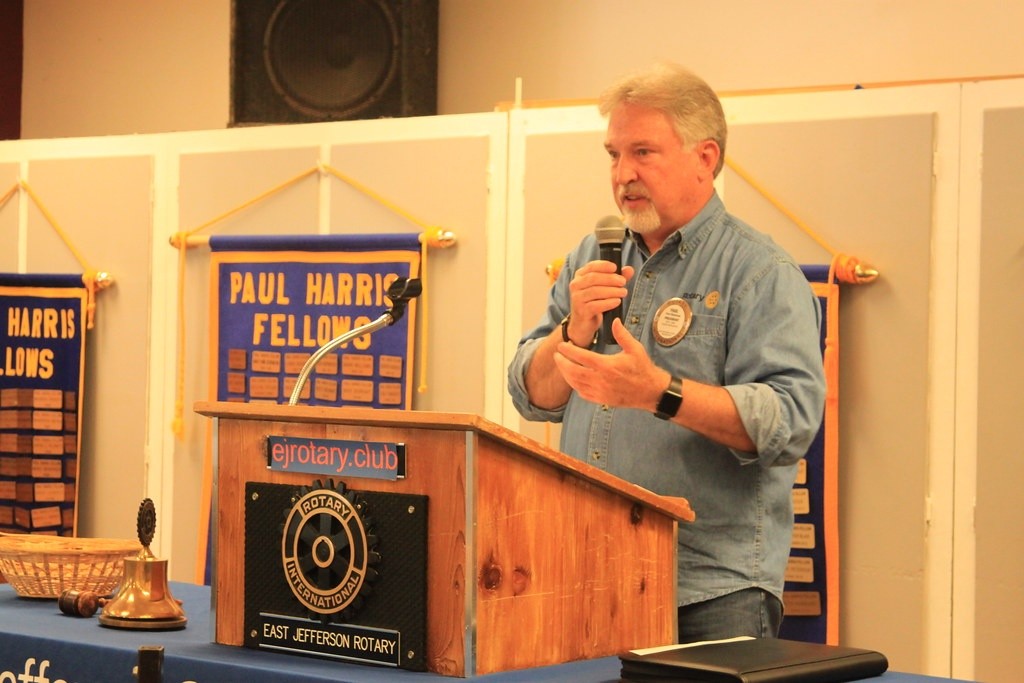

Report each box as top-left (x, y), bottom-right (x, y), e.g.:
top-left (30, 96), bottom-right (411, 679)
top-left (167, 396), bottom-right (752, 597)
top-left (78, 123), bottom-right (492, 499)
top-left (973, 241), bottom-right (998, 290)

top-left (561, 313), bottom-right (598, 350)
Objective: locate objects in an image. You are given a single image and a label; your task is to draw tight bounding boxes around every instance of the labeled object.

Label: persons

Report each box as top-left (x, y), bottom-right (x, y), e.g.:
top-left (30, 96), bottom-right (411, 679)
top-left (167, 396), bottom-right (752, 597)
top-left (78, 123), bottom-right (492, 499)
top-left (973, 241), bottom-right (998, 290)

top-left (508, 63), bottom-right (826, 644)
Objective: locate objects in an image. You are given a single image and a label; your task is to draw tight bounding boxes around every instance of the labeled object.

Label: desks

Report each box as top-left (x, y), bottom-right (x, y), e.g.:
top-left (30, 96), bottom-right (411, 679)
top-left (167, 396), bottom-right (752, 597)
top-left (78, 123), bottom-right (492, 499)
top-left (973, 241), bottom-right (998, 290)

top-left (1, 577), bottom-right (978, 681)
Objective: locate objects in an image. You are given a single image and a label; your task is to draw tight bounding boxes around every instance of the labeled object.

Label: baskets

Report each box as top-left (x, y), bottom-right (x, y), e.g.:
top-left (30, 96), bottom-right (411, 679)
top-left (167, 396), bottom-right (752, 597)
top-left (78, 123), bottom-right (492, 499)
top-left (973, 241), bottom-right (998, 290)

top-left (0, 535), bottom-right (147, 598)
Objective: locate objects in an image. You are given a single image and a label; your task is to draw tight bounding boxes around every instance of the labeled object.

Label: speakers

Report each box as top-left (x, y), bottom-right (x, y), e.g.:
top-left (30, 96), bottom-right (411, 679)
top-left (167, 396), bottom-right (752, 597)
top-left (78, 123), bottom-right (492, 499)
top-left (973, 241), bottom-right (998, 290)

top-left (227, 1), bottom-right (439, 130)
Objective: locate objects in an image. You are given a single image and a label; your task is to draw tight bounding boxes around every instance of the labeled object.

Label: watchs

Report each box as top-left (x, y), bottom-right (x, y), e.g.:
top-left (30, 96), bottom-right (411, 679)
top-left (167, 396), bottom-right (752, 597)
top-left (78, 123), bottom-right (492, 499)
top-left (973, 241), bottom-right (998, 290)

top-left (653, 376), bottom-right (683, 421)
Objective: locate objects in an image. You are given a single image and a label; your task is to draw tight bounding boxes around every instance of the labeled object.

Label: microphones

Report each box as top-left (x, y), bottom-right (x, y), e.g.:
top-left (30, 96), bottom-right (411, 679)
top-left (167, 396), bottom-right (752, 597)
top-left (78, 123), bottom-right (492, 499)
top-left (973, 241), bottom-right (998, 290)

top-left (595, 214), bottom-right (625, 347)
